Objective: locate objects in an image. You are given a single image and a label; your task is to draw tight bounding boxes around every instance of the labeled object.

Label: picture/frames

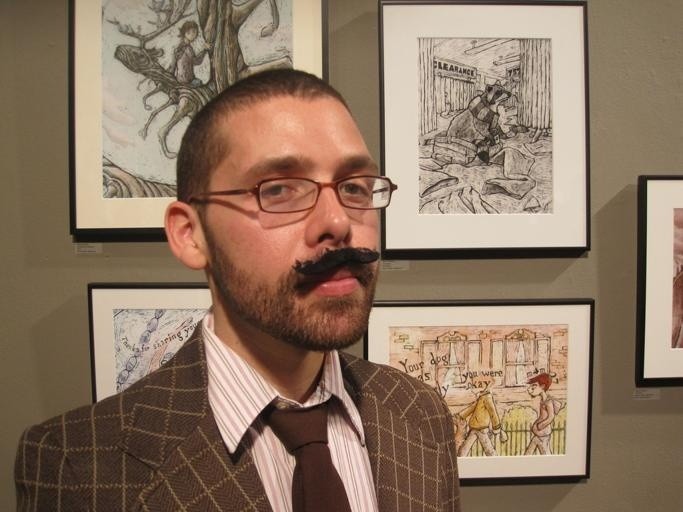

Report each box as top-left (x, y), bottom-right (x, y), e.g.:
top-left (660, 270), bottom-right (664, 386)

top-left (635, 173), bottom-right (683, 388)
top-left (377, 1), bottom-right (591, 253)
top-left (363, 297), bottom-right (595, 487)
top-left (87, 281), bottom-right (210, 404)
top-left (67, 0), bottom-right (330, 237)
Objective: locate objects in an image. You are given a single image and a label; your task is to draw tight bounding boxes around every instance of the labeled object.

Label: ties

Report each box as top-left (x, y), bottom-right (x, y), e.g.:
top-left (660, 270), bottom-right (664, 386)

top-left (264, 405), bottom-right (351, 512)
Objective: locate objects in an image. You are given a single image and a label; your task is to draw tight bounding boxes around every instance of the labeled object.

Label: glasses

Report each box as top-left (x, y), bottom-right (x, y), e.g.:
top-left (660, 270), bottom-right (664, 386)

top-left (186, 175), bottom-right (397, 213)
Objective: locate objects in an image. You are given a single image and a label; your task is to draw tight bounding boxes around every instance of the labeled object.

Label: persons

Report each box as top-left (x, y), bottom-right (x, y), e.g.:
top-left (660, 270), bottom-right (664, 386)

top-left (14, 66), bottom-right (463, 511)
